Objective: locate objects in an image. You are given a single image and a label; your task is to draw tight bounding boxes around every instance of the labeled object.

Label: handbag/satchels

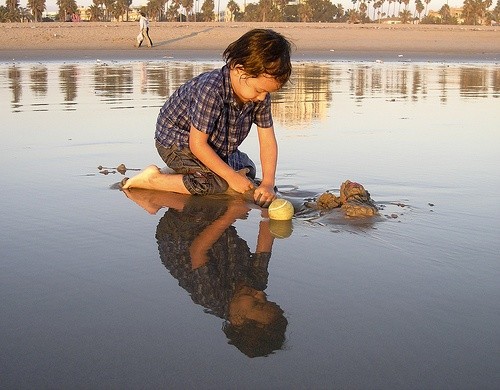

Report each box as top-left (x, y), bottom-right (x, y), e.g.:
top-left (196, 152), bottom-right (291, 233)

top-left (137, 31), bottom-right (144, 43)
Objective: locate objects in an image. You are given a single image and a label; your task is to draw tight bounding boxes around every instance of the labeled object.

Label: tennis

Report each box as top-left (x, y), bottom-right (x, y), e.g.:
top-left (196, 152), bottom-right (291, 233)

top-left (267, 198), bottom-right (293, 220)
top-left (268, 220), bottom-right (293, 238)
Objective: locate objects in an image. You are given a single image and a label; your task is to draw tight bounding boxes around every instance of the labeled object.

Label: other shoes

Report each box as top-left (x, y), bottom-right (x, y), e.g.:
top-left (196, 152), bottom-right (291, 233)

top-left (146, 45), bottom-right (152, 48)
top-left (133, 45), bottom-right (139, 48)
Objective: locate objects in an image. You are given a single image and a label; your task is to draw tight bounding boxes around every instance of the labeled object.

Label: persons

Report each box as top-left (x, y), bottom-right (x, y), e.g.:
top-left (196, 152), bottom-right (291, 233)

top-left (117, 28), bottom-right (297, 210)
top-left (64, 5), bottom-right (80, 23)
top-left (121, 188), bottom-right (287, 357)
top-left (132, 11), bottom-right (153, 48)
